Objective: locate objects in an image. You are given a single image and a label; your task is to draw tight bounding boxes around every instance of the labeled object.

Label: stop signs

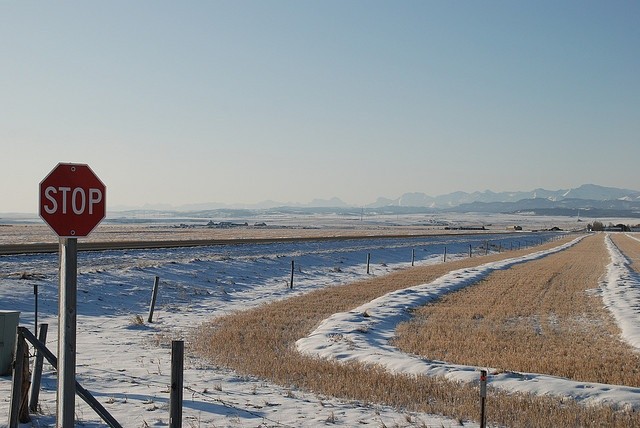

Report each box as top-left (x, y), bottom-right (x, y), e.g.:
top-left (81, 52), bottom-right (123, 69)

top-left (39, 161), bottom-right (106, 237)
